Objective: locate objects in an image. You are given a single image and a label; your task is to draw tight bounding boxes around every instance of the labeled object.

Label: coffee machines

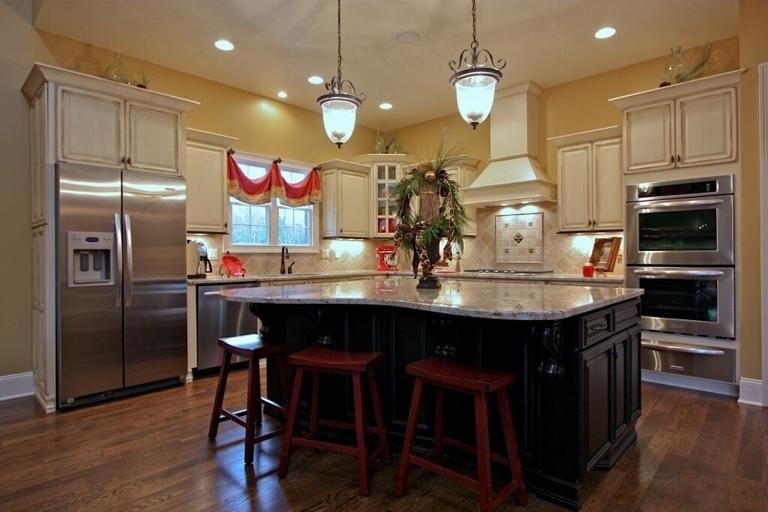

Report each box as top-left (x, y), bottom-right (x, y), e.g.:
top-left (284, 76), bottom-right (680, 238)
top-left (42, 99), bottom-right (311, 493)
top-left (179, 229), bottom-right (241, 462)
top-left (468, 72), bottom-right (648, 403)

top-left (187, 240), bottom-right (214, 279)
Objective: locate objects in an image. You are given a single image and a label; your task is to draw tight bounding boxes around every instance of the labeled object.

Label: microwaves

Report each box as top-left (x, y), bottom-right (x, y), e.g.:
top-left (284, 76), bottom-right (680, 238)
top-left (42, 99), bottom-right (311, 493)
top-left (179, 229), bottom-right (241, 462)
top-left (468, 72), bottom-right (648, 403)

top-left (463, 267), bottom-right (554, 274)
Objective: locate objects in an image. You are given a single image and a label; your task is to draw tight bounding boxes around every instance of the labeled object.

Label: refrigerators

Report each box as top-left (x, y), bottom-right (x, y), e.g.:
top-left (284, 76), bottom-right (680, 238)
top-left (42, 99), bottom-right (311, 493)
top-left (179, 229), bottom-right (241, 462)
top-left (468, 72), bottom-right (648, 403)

top-left (54, 161), bottom-right (189, 409)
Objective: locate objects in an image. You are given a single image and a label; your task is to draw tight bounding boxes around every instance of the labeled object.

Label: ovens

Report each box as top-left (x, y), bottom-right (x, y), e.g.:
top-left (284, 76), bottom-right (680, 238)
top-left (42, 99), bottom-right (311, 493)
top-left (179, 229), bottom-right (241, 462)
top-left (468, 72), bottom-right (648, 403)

top-left (625, 172), bottom-right (738, 386)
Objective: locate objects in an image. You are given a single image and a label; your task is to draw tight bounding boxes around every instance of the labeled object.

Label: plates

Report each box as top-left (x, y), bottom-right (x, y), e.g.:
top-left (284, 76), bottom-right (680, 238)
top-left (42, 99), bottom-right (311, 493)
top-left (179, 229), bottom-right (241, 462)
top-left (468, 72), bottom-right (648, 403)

top-left (222, 255), bottom-right (244, 276)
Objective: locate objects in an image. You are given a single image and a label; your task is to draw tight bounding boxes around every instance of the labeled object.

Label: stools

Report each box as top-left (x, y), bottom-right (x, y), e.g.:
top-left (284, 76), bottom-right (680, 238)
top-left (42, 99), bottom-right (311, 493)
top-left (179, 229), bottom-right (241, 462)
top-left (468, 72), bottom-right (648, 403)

top-left (391, 357), bottom-right (528, 512)
top-left (276, 346), bottom-right (392, 498)
top-left (205, 331), bottom-right (301, 466)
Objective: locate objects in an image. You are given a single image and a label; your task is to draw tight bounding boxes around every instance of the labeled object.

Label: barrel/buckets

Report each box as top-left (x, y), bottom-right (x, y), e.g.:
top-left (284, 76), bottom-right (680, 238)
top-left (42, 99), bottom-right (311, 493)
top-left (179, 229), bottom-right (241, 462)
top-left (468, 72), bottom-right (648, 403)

top-left (376, 244), bottom-right (398, 271)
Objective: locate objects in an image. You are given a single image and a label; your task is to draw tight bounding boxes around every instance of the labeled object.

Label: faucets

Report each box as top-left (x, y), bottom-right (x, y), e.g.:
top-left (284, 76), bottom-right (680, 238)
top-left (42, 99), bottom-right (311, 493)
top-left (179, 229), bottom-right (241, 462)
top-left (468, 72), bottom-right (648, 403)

top-left (279, 245), bottom-right (290, 274)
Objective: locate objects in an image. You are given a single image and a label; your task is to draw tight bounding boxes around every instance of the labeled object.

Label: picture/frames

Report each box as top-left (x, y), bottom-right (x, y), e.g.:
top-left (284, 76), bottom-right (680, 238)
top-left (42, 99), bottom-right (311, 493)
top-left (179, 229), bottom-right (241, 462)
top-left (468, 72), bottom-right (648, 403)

top-left (586, 235), bottom-right (622, 273)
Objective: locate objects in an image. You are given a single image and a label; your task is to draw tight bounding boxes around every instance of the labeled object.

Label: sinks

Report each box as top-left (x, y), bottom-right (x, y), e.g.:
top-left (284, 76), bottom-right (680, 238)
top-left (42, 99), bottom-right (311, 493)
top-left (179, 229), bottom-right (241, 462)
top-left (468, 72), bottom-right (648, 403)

top-left (271, 272), bottom-right (318, 277)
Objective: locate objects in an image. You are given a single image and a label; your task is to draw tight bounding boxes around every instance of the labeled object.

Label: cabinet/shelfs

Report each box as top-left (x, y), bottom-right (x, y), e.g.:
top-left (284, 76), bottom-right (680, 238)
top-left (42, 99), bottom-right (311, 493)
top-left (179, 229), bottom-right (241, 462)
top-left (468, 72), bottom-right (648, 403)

top-left (574, 298), bottom-right (641, 504)
top-left (309, 274), bottom-right (344, 284)
top-left (386, 273), bottom-right (402, 280)
top-left (344, 273), bottom-right (373, 282)
top-left (318, 160), bottom-right (368, 239)
top-left (257, 278), bottom-right (308, 369)
top-left (20, 59), bottom-right (202, 177)
top-left (545, 276), bottom-right (624, 288)
top-left (607, 65), bottom-right (750, 184)
top-left (367, 153), bottom-right (410, 240)
top-left (488, 275), bottom-right (544, 284)
top-left (402, 273), bottom-right (447, 280)
top-left (373, 274), bottom-right (386, 280)
top-left (401, 154), bottom-right (482, 238)
top-left (447, 275), bottom-right (489, 282)
top-left (545, 123), bottom-right (625, 235)
top-left (184, 126), bottom-right (239, 236)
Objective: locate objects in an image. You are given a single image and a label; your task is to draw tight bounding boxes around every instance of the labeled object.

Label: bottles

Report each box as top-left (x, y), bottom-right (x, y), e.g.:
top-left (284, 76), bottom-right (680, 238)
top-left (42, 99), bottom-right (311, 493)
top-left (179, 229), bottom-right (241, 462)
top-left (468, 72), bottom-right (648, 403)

top-left (666, 46), bottom-right (688, 84)
top-left (104, 50), bottom-right (133, 84)
top-left (582, 263), bottom-right (593, 276)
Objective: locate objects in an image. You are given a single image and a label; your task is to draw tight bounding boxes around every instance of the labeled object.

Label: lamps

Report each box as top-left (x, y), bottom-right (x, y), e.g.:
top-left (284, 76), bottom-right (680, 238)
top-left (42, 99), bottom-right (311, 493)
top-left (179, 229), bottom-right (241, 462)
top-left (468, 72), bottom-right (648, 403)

top-left (445, 1), bottom-right (505, 130)
top-left (315, 1), bottom-right (368, 149)
